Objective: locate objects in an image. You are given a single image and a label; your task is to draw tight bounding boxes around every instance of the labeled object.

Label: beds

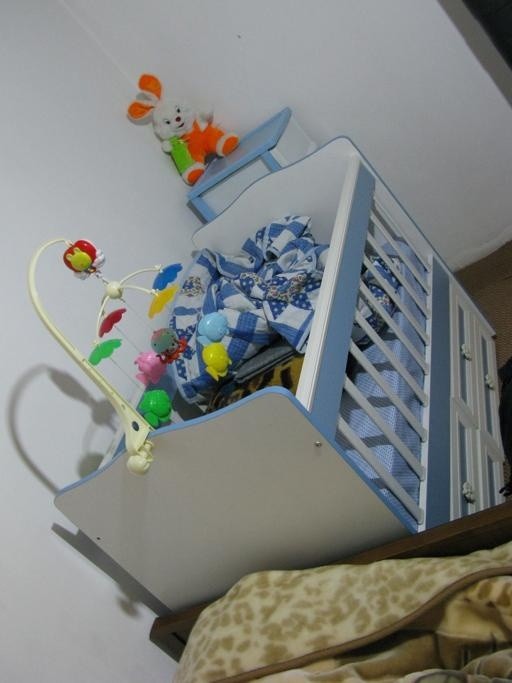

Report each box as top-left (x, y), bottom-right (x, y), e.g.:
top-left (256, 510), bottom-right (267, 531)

top-left (54, 136), bottom-right (507, 616)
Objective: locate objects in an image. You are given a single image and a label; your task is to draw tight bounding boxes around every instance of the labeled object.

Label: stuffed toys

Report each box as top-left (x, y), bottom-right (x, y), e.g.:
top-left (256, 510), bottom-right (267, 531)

top-left (126, 73), bottom-right (240, 186)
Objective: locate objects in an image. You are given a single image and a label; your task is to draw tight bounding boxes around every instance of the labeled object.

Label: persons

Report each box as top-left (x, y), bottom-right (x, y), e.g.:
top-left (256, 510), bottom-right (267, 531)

top-left (63, 237), bottom-right (231, 429)
